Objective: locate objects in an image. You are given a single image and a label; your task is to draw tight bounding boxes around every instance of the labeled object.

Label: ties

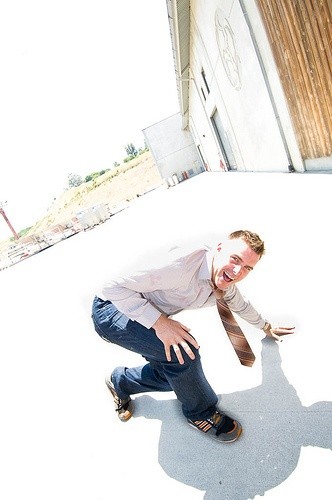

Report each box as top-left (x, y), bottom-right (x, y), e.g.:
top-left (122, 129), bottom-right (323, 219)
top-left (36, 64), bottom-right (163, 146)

top-left (208, 279), bottom-right (256, 367)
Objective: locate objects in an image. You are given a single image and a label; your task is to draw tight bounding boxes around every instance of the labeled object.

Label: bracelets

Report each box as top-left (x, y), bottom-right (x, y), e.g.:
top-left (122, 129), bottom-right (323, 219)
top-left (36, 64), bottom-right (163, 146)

top-left (263, 322), bottom-right (271, 331)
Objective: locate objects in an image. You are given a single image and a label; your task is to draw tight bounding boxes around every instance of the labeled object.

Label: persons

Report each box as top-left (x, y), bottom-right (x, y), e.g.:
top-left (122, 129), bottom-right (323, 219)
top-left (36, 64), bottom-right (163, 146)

top-left (91, 230), bottom-right (296, 444)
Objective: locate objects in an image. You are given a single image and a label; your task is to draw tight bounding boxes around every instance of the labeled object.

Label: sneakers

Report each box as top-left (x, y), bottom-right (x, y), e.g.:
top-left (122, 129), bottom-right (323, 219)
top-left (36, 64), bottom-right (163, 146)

top-left (186, 405), bottom-right (242, 443)
top-left (105, 370), bottom-right (135, 420)
